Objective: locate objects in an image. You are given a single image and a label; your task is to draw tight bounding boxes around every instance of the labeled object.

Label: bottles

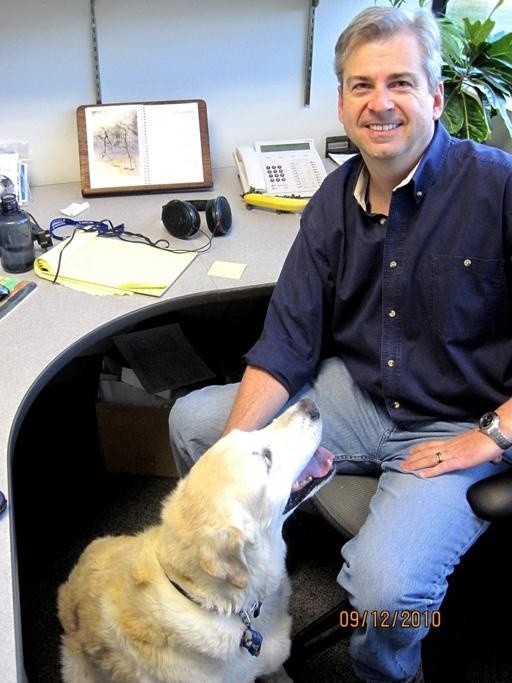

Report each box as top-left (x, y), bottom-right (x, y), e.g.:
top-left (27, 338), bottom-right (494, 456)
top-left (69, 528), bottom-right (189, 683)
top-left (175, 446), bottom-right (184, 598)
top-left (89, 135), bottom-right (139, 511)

top-left (1, 193), bottom-right (36, 273)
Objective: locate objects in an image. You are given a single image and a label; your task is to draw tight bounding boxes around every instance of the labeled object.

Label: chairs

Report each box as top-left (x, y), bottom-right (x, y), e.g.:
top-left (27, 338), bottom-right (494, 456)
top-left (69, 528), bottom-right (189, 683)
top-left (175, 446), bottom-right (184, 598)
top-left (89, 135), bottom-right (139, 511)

top-left (283, 466), bottom-right (512, 667)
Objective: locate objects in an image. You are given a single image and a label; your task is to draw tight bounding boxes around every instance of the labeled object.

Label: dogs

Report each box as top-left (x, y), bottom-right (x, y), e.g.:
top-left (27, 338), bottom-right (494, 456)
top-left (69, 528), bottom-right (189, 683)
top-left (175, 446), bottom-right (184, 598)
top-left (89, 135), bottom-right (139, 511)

top-left (51, 396), bottom-right (338, 683)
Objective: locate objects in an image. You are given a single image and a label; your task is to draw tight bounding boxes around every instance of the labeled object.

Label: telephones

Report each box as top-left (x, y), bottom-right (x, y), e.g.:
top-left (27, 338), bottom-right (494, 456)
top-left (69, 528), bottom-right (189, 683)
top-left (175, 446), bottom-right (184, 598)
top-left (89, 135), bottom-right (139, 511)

top-left (232, 139), bottom-right (329, 200)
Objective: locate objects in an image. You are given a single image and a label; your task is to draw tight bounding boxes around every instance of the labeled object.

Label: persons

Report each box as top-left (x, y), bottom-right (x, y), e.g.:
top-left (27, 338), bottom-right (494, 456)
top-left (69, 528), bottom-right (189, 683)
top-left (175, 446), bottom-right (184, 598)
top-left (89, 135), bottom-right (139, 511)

top-left (165, 3), bottom-right (512, 683)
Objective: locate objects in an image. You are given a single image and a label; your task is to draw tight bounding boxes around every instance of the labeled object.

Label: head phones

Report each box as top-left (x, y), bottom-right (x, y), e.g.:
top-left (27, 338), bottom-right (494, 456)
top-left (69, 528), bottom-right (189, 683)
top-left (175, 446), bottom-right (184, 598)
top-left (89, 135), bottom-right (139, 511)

top-left (162, 196), bottom-right (232, 240)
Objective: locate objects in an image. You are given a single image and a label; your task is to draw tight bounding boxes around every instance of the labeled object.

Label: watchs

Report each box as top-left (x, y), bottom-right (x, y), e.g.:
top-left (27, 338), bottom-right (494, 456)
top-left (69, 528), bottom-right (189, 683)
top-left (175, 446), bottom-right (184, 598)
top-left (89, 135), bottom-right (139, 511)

top-left (478, 409), bottom-right (512, 450)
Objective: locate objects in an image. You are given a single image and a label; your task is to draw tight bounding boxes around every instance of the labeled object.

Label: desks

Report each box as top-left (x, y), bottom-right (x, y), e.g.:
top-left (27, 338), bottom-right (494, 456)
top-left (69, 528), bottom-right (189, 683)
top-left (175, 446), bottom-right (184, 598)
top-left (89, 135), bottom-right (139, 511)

top-left (0, 159), bottom-right (341, 683)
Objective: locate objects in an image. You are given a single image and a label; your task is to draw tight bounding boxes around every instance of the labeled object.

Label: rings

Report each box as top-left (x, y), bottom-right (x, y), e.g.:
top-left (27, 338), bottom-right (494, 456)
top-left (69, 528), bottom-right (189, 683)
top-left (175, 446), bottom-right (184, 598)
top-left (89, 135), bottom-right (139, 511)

top-left (437, 451), bottom-right (442, 463)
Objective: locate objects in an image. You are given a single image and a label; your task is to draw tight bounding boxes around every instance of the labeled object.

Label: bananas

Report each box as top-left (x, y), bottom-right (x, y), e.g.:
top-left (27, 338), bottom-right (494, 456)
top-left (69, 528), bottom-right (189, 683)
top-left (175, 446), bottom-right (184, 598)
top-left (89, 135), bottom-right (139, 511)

top-left (243, 193), bottom-right (313, 212)
